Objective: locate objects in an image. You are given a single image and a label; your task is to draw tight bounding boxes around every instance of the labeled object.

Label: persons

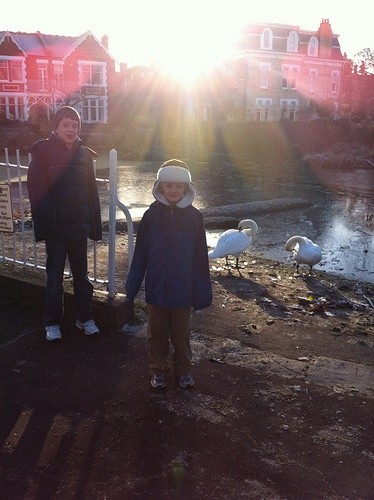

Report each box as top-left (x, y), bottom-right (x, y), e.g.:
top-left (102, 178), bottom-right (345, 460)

top-left (27, 105), bottom-right (104, 341)
top-left (124, 160), bottom-right (213, 390)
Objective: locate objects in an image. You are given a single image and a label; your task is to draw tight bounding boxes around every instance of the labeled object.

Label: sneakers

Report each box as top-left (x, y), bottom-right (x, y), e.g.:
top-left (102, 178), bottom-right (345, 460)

top-left (178, 372), bottom-right (195, 387)
top-left (44, 325), bottom-right (62, 341)
top-left (76, 319), bottom-right (99, 336)
top-left (151, 374), bottom-right (167, 388)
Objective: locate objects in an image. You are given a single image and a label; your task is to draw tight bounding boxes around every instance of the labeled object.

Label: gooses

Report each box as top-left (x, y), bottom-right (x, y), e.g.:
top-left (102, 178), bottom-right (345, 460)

top-left (208, 218), bottom-right (258, 270)
top-left (284, 236), bottom-right (322, 278)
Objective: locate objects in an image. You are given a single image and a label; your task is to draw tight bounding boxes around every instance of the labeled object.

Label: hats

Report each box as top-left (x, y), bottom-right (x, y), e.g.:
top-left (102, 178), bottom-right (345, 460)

top-left (151, 158), bottom-right (197, 210)
